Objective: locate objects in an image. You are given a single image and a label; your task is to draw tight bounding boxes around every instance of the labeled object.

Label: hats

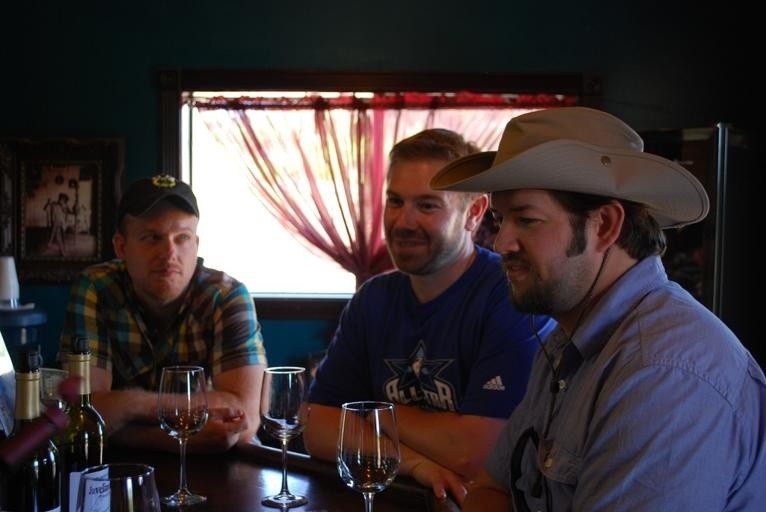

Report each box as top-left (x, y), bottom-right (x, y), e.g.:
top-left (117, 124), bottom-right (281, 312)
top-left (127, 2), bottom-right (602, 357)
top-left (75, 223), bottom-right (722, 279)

top-left (429, 104), bottom-right (710, 229)
top-left (120, 173), bottom-right (199, 219)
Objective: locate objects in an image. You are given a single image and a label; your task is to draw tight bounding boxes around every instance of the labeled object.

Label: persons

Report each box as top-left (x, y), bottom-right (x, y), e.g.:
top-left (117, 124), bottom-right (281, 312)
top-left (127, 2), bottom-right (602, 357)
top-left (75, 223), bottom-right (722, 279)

top-left (295, 126), bottom-right (560, 504)
top-left (427, 106), bottom-right (766, 511)
top-left (54, 171), bottom-right (271, 455)
top-left (46, 192), bottom-right (71, 257)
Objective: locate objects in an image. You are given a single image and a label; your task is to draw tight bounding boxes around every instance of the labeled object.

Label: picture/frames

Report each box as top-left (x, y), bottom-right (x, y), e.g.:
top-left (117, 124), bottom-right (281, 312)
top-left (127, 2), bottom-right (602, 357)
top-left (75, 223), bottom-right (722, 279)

top-left (0, 136), bottom-right (125, 284)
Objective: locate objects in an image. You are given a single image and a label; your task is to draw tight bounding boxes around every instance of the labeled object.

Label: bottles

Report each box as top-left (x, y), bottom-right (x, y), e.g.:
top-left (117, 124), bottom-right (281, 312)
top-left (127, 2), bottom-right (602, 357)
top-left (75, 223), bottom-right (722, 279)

top-left (61, 334), bottom-right (111, 512)
top-left (0, 342), bottom-right (60, 512)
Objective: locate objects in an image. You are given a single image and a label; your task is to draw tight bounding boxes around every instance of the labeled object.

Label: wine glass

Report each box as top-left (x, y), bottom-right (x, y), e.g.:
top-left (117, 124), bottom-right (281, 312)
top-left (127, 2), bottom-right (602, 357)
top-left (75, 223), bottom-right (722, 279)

top-left (157, 365), bottom-right (210, 506)
top-left (336, 401), bottom-right (402, 512)
top-left (259, 366), bottom-right (311, 508)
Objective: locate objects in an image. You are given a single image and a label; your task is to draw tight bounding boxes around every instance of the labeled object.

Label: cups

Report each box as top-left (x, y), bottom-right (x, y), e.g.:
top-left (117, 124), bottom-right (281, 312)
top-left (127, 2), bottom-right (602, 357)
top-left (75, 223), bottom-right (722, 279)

top-left (77, 462), bottom-right (162, 512)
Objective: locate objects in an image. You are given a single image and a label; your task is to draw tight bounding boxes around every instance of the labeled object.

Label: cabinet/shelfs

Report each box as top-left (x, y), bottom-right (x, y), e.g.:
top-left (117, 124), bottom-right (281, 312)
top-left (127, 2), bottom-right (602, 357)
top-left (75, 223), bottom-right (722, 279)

top-left (653, 122), bottom-right (765, 367)
